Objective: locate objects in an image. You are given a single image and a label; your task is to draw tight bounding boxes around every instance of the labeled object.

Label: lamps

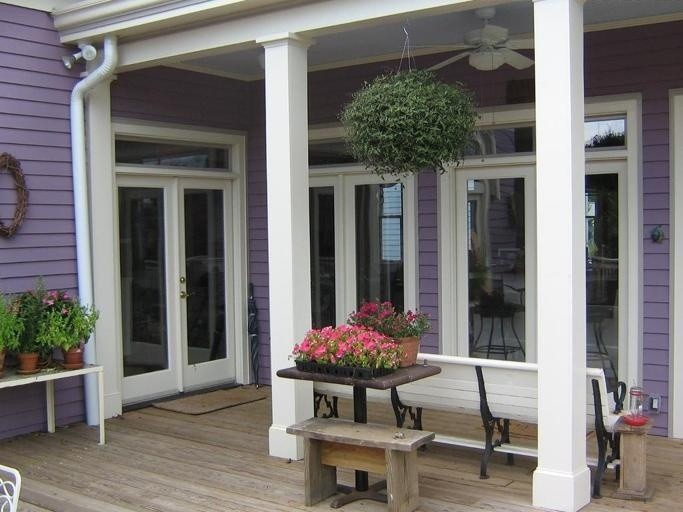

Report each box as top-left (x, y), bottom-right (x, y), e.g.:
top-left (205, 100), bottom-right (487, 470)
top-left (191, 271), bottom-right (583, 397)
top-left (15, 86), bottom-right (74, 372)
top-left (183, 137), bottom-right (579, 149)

top-left (63, 45), bottom-right (96, 68)
top-left (470, 51), bottom-right (505, 72)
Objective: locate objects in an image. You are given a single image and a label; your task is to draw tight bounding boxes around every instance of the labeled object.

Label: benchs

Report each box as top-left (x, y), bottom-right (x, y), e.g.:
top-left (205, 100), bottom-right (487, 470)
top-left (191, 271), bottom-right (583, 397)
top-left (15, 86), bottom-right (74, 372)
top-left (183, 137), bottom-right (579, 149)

top-left (313, 349), bottom-right (626, 500)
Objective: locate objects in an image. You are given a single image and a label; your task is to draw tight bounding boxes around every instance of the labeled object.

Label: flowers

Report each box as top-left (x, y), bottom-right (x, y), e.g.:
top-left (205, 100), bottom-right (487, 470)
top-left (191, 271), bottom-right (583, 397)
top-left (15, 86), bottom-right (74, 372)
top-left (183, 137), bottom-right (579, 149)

top-left (292, 299), bottom-right (429, 369)
top-left (0, 287), bottom-right (101, 353)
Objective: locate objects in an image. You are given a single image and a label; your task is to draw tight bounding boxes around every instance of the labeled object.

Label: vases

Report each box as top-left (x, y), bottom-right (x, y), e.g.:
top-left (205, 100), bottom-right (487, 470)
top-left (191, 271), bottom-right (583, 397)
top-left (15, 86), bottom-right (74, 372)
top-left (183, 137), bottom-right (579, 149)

top-left (294, 334), bottom-right (421, 379)
top-left (14, 348), bottom-right (85, 375)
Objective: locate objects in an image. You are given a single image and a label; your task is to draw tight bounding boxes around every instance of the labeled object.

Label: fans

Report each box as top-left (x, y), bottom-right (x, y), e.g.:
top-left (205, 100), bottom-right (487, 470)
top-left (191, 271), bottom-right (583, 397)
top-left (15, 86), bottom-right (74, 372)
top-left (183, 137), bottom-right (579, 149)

top-left (417, 13), bottom-right (535, 72)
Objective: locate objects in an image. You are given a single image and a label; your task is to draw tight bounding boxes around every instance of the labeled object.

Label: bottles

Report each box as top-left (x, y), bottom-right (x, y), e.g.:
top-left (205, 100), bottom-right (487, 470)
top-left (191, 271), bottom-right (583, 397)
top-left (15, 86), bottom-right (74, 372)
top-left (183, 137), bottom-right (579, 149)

top-left (628, 386), bottom-right (645, 421)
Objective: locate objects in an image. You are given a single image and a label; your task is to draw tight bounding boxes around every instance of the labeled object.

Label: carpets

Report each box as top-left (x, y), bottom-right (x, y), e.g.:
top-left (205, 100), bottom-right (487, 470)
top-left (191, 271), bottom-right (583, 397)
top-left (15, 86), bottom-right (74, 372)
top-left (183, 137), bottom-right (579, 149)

top-left (152, 386), bottom-right (262, 415)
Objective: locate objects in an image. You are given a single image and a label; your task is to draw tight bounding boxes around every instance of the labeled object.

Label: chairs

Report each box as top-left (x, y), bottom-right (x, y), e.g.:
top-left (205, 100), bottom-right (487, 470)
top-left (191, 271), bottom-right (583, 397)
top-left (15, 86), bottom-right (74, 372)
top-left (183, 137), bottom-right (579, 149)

top-left (473, 278), bottom-right (525, 361)
top-left (0, 464), bottom-right (22, 512)
top-left (579, 279), bottom-right (616, 372)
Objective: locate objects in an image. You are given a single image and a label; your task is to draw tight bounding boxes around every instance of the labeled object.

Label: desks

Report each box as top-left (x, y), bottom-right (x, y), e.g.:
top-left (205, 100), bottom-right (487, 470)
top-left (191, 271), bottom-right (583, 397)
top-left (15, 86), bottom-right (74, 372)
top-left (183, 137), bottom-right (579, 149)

top-left (275, 364), bottom-right (448, 510)
top-left (0, 360), bottom-right (105, 445)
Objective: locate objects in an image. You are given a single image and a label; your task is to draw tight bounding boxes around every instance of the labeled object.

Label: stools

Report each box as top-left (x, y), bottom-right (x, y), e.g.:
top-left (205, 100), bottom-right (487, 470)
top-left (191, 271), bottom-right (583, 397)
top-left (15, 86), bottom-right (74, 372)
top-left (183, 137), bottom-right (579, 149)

top-left (286, 411), bottom-right (433, 511)
